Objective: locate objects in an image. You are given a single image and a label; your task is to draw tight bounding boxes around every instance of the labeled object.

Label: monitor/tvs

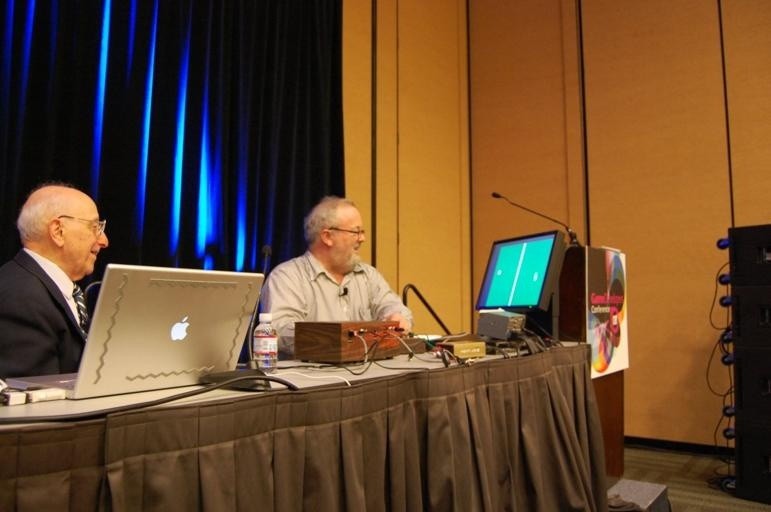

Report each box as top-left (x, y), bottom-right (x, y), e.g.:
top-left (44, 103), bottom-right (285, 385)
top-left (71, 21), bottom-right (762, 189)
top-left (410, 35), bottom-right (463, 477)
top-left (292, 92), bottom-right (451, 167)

top-left (475, 229), bottom-right (568, 343)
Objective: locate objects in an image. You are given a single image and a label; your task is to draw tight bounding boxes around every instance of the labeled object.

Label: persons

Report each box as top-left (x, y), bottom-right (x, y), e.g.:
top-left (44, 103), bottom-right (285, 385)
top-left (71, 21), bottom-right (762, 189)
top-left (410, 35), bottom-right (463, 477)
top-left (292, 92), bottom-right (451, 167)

top-left (260, 196), bottom-right (413, 361)
top-left (0, 184), bottom-right (111, 379)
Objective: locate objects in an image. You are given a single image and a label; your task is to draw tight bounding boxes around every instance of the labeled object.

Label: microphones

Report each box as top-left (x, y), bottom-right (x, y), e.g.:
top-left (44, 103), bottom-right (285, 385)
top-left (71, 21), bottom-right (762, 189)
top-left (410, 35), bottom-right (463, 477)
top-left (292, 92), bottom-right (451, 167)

top-left (491, 190), bottom-right (582, 250)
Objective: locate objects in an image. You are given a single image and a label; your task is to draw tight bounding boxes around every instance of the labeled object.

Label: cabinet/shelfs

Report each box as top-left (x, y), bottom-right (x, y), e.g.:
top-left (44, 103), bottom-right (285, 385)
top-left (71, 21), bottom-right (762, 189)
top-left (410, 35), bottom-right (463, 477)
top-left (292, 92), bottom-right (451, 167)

top-left (471, 0), bottom-right (587, 336)
top-left (586, 0), bottom-right (733, 450)
top-left (342, 0), bottom-right (471, 335)
top-left (723, 0), bottom-right (771, 224)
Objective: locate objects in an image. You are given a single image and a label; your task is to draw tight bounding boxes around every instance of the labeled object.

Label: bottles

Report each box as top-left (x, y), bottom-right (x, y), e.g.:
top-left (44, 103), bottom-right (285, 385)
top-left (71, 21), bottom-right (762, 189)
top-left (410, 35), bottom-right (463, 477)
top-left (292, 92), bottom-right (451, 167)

top-left (253, 313), bottom-right (278, 374)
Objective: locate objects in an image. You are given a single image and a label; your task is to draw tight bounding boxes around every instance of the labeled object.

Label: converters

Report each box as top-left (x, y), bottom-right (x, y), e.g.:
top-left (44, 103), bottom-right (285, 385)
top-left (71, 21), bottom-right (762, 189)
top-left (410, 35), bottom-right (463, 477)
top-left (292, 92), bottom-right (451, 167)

top-left (436, 340), bottom-right (486, 358)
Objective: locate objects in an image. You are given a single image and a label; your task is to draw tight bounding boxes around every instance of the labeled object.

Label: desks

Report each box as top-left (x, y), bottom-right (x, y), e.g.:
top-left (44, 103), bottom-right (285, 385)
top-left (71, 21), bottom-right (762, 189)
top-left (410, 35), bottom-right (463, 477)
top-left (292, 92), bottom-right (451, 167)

top-left (0, 333), bottom-right (608, 512)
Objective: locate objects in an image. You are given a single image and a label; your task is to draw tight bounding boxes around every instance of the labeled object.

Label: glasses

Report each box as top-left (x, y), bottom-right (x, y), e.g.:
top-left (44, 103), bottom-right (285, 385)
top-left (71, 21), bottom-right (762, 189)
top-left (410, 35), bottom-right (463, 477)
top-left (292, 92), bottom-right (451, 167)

top-left (57, 215), bottom-right (107, 237)
top-left (329, 226), bottom-right (365, 238)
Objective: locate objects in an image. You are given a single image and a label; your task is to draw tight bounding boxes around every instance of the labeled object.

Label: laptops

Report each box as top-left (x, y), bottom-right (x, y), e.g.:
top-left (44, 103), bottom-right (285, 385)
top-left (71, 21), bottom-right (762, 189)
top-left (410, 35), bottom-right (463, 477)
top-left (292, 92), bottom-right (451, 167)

top-left (5, 260), bottom-right (266, 404)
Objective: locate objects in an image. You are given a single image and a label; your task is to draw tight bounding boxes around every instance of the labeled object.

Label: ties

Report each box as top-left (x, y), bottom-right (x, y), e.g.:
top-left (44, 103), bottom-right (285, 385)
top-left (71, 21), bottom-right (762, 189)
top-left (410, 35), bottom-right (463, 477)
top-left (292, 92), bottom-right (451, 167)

top-left (72, 282), bottom-right (90, 334)
